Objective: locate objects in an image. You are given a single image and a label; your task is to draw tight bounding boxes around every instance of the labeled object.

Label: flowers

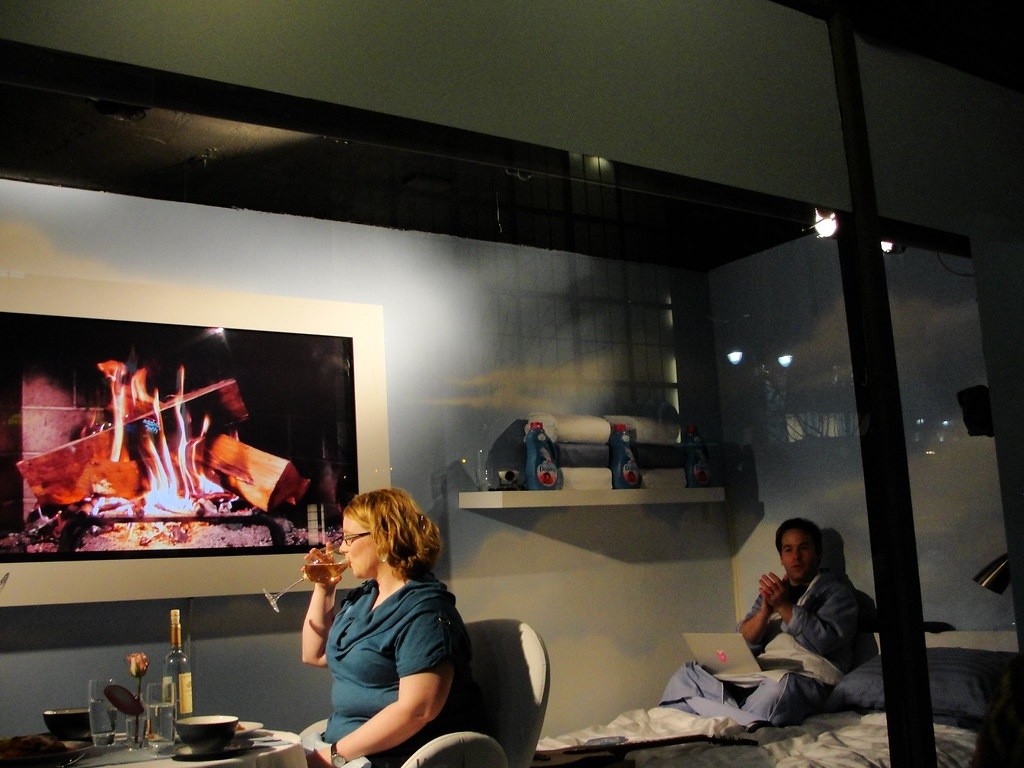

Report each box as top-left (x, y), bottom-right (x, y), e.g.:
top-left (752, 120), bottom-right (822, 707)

top-left (126, 652), bottom-right (150, 742)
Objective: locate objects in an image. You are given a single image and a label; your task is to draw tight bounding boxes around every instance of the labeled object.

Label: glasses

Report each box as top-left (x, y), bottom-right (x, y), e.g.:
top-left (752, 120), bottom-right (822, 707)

top-left (345, 531), bottom-right (371, 546)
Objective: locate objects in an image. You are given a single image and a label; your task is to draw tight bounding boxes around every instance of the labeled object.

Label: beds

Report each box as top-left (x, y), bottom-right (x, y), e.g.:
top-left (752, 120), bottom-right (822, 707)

top-left (532, 629), bottom-right (1024, 768)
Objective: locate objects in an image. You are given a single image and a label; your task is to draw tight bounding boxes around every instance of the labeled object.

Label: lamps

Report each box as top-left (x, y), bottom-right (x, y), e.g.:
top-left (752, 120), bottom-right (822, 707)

top-left (972, 552), bottom-right (1012, 595)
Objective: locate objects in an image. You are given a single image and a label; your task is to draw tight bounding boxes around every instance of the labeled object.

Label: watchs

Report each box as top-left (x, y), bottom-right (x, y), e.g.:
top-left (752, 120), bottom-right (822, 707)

top-left (331, 742), bottom-right (346, 768)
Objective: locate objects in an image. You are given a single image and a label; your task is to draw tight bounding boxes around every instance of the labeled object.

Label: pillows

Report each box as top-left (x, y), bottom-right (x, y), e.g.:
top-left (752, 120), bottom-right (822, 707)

top-left (825, 639), bottom-right (1021, 722)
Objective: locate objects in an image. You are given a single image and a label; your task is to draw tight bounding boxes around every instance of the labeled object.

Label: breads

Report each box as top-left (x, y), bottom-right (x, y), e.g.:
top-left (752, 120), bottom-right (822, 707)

top-left (0, 735), bottom-right (72, 757)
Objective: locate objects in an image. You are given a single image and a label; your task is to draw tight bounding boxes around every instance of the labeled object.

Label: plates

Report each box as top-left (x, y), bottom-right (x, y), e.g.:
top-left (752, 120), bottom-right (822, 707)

top-left (0, 741), bottom-right (94, 768)
top-left (235, 722), bottom-right (263, 735)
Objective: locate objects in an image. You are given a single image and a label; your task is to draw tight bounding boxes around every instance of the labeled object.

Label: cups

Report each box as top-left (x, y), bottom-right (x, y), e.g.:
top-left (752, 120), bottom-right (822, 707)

top-left (476, 449), bottom-right (494, 491)
top-left (147, 682), bottom-right (178, 757)
top-left (88, 679), bottom-right (117, 748)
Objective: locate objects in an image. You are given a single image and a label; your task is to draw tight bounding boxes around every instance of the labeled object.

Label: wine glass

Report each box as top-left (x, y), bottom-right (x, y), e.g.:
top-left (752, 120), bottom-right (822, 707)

top-left (263, 541), bottom-right (351, 612)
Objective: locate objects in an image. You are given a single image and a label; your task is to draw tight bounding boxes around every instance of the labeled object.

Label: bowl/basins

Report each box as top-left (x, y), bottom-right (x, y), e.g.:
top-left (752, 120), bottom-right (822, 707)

top-left (175, 714), bottom-right (239, 754)
top-left (45, 707), bottom-right (117, 739)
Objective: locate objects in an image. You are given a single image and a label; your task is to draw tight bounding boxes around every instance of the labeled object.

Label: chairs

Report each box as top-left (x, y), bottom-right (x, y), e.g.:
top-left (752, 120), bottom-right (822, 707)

top-left (303, 618), bottom-right (550, 768)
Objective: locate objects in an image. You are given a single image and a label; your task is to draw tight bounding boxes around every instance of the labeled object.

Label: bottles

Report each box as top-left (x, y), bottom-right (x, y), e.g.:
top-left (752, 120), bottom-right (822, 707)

top-left (609, 424), bottom-right (640, 489)
top-left (526, 422), bottom-right (557, 491)
top-left (162, 625), bottom-right (192, 720)
top-left (685, 425), bottom-right (712, 487)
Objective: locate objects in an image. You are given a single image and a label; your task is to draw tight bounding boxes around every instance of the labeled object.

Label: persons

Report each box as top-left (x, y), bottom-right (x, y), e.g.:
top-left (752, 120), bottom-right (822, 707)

top-left (659, 517), bottom-right (862, 733)
top-left (301, 488), bottom-right (475, 768)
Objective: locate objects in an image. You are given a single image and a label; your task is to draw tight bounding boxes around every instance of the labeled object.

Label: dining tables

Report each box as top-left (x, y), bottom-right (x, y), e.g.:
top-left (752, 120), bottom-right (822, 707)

top-left (0, 728), bottom-right (309, 768)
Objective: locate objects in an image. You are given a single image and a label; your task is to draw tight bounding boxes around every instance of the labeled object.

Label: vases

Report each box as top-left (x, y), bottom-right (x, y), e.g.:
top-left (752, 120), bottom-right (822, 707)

top-left (124, 691), bottom-right (150, 750)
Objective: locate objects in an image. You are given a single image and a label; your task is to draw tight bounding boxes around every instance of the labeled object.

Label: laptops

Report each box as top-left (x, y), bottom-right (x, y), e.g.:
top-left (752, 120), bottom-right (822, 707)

top-left (683, 633), bottom-right (800, 675)
